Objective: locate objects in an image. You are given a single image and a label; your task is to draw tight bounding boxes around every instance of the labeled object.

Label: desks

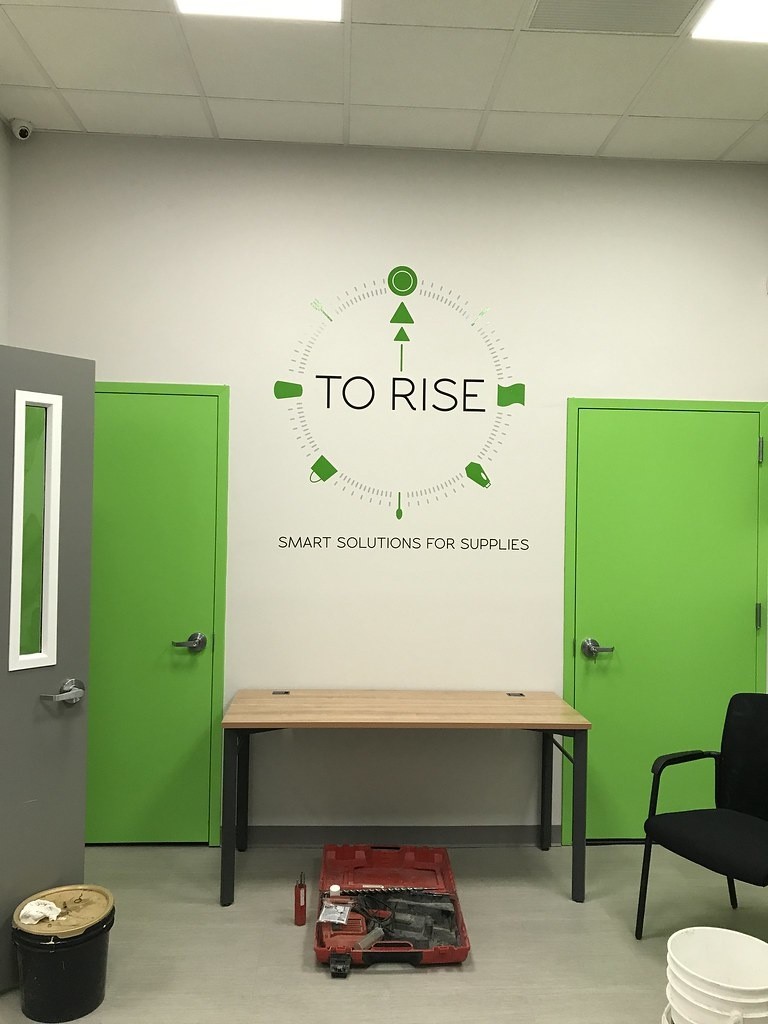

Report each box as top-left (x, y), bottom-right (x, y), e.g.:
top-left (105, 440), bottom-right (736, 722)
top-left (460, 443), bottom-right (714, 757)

top-left (218, 689), bottom-right (593, 904)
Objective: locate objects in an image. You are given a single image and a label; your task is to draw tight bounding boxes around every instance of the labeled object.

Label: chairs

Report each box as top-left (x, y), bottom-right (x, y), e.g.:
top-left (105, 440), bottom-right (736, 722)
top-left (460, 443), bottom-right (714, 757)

top-left (634, 693), bottom-right (768, 940)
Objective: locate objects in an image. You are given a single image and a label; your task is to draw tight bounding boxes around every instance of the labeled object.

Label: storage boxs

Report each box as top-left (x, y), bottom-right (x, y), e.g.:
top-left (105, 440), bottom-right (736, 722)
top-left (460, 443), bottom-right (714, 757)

top-left (314, 842), bottom-right (471, 968)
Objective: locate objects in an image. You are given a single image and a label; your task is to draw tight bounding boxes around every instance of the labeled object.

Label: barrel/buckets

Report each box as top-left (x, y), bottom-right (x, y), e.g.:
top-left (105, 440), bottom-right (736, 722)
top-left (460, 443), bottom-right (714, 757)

top-left (12, 885), bottom-right (116, 1023)
top-left (661, 926), bottom-right (768, 1024)
